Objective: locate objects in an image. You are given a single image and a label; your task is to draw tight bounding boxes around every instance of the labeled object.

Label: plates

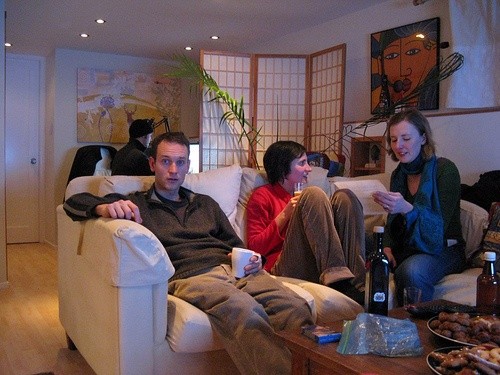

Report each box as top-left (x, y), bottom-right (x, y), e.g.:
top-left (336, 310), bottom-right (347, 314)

top-left (426, 347), bottom-right (473, 375)
top-left (426, 312), bottom-right (500, 347)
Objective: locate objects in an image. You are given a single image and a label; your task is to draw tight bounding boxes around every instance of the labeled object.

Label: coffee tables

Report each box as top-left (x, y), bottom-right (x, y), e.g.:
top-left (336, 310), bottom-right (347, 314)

top-left (274, 298), bottom-right (500, 375)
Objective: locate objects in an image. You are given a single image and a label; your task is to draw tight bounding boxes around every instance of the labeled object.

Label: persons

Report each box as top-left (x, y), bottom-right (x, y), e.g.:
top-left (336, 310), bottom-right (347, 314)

top-left (111, 119), bottom-right (155, 176)
top-left (246, 140), bottom-right (366, 307)
top-left (63, 132), bottom-right (314, 375)
top-left (371, 108), bottom-right (466, 303)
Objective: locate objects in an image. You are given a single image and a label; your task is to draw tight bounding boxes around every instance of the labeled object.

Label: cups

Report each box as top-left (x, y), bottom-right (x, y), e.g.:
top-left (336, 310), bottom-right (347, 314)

top-left (294, 182), bottom-right (304, 200)
top-left (404, 286), bottom-right (422, 310)
top-left (231, 247), bottom-right (261, 279)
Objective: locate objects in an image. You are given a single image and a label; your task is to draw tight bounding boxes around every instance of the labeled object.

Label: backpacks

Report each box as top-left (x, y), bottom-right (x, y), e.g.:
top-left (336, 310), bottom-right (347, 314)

top-left (479, 170), bottom-right (500, 212)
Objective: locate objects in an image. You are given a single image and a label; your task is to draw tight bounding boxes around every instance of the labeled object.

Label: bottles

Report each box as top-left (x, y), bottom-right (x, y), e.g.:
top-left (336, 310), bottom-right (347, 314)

top-left (476, 251), bottom-right (500, 318)
top-left (379, 75), bottom-right (390, 119)
top-left (364, 226), bottom-right (389, 317)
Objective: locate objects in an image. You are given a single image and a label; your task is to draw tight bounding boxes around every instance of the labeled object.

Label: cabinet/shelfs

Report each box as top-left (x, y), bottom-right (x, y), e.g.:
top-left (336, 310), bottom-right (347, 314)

top-left (349, 134), bottom-right (387, 179)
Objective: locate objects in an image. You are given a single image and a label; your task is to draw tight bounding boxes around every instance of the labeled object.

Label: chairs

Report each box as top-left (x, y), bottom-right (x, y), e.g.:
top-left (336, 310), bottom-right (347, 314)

top-left (62, 144), bottom-right (118, 205)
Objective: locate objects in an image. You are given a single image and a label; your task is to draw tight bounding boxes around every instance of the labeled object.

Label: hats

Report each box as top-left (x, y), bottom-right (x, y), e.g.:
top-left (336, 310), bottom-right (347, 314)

top-left (129, 119), bottom-right (153, 138)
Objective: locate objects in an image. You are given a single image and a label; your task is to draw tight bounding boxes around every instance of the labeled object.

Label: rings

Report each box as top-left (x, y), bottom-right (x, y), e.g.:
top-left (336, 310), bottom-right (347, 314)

top-left (383, 205), bottom-right (388, 209)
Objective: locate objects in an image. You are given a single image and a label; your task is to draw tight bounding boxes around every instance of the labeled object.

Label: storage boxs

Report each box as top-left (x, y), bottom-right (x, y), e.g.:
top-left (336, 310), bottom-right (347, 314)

top-left (301, 322), bottom-right (342, 345)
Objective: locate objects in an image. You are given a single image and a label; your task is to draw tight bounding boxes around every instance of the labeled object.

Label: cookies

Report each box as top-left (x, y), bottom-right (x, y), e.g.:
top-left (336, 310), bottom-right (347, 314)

top-left (429, 311), bottom-right (500, 346)
top-left (431, 345), bottom-right (500, 375)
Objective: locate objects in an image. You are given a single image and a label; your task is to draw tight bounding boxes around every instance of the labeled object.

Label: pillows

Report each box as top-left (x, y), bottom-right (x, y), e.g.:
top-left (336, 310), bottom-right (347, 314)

top-left (97, 162), bottom-right (243, 226)
top-left (459, 200), bottom-right (490, 258)
top-left (232, 166), bottom-right (330, 250)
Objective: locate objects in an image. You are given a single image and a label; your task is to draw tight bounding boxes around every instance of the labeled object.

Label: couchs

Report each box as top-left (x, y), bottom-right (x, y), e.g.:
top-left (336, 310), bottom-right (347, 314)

top-left (55, 163), bottom-right (500, 375)
